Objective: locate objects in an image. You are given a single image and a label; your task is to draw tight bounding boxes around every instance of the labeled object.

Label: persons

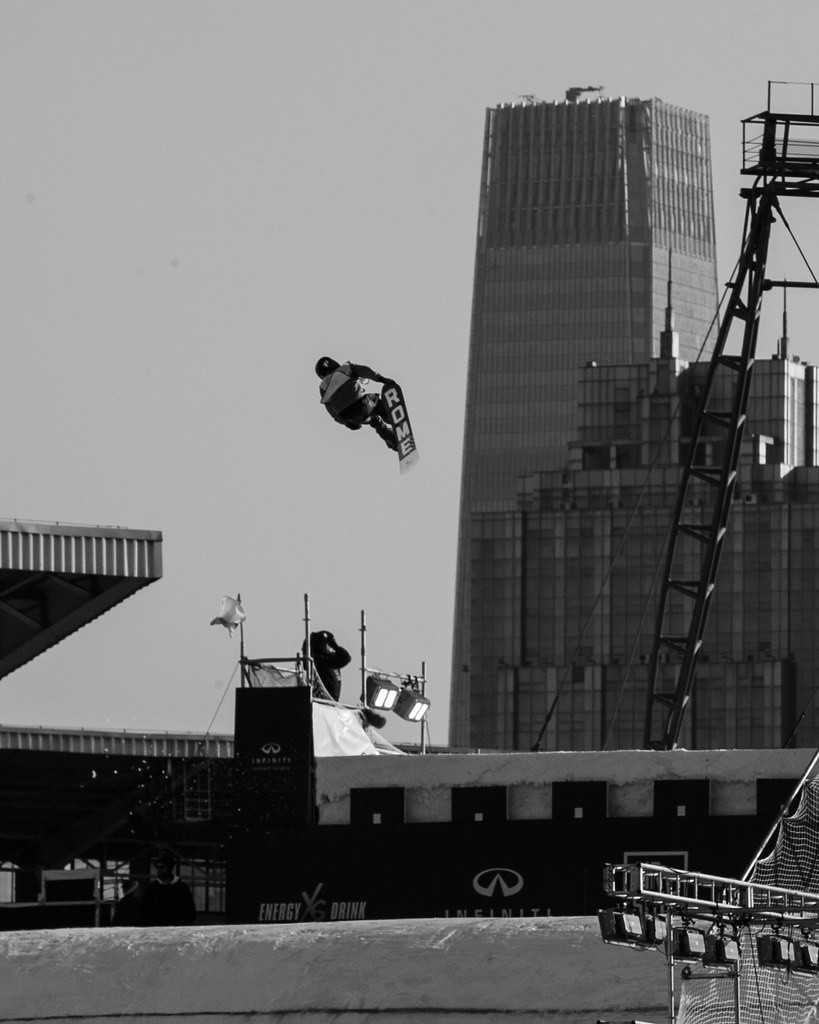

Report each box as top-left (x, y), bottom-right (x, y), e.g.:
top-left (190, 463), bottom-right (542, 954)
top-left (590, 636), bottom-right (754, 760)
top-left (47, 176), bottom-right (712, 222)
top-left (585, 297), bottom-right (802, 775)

top-left (315, 357), bottom-right (401, 452)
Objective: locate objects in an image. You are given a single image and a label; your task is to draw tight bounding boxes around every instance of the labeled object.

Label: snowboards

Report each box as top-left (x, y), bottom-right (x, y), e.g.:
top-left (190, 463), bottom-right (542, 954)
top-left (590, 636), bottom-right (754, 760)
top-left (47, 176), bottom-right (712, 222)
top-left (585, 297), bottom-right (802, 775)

top-left (381, 382), bottom-right (420, 475)
